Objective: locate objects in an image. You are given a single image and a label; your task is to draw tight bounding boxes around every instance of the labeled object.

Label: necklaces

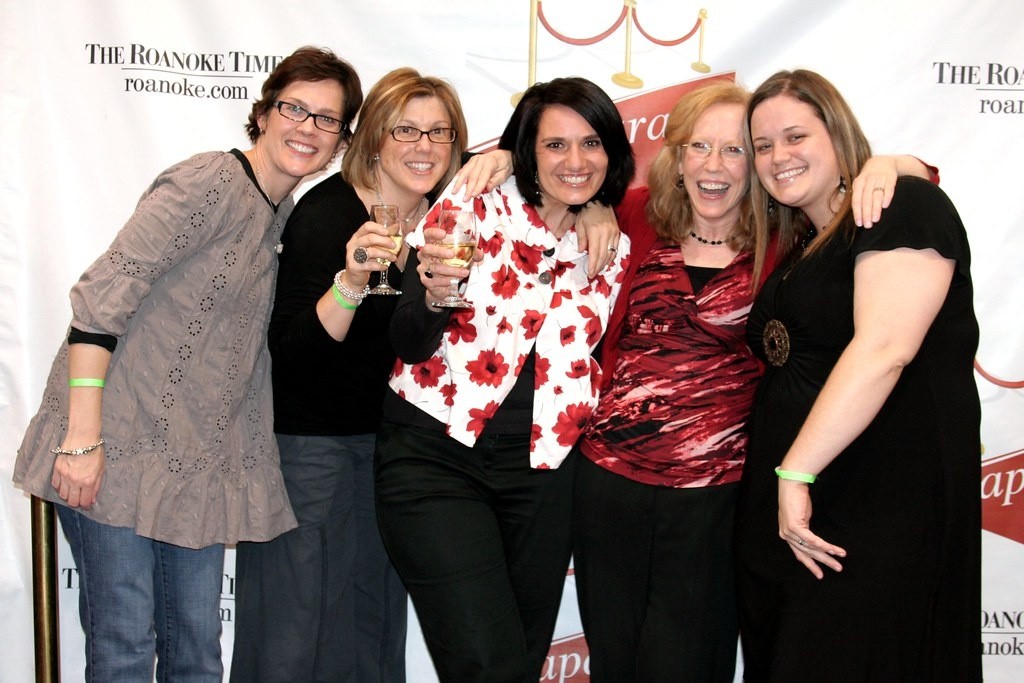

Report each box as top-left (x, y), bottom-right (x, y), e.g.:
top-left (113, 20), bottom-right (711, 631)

top-left (375, 189), bottom-right (421, 223)
top-left (257, 170), bottom-right (283, 254)
top-left (689, 231), bottom-right (726, 245)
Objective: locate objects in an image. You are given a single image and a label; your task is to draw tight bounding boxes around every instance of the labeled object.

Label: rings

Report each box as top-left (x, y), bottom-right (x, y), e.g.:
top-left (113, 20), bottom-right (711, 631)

top-left (873, 187), bottom-right (885, 192)
top-left (424, 263), bottom-right (434, 279)
top-left (608, 245), bottom-right (617, 253)
top-left (799, 539), bottom-right (805, 545)
top-left (353, 247), bottom-right (369, 264)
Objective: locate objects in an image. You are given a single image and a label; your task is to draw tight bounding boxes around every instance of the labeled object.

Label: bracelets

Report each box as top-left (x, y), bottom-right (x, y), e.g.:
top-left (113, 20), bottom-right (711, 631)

top-left (331, 283), bottom-right (362, 310)
top-left (68, 378), bottom-right (105, 388)
top-left (334, 269), bottom-right (370, 300)
top-left (774, 466), bottom-right (816, 483)
top-left (50, 439), bottom-right (104, 455)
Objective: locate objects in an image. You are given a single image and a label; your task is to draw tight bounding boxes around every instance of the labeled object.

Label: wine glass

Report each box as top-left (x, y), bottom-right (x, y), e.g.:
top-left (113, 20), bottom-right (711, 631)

top-left (432, 210), bottom-right (478, 308)
top-left (365, 204), bottom-right (404, 295)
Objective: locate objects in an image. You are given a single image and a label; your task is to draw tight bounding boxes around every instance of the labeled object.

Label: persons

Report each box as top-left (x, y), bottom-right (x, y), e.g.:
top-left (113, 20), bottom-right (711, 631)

top-left (227, 68), bottom-right (620, 683)
top-left (373, 77), bottom-right (630, 683)
top-left (452, 84), bottom-right (940, 683)
top-left (741, 70), bottom-right (979, 683)
top-left (13, 44), bottom-right (364, 683)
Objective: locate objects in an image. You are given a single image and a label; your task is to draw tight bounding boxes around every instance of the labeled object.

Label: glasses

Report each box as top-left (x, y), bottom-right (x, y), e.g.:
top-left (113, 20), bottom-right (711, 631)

top-left (678, 141), bottom-right (754, 163)
top-left (381, 126), bottom-right (458, 143)
top-left (270, 101), bottom-right (351, 134)
